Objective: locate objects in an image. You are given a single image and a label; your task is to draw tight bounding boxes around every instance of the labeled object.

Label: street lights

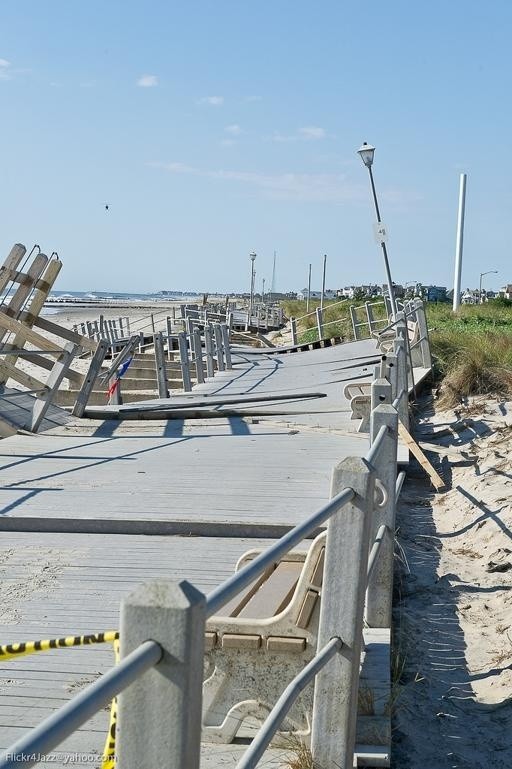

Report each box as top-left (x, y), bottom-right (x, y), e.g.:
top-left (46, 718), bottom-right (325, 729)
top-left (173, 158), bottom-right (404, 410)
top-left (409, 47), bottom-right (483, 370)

top-left (252, 269), bottom-right (256, 305)
top-left (261, 278), bottom-right (266, 302)
top-left (248, 251), bottom-right (256, 322)
top-left (354, 140), bottom-right (408, 344)
top-left (479, 270), bottom-right (499, 304)
top-left (402, 280), bottom-right (418, 300)
